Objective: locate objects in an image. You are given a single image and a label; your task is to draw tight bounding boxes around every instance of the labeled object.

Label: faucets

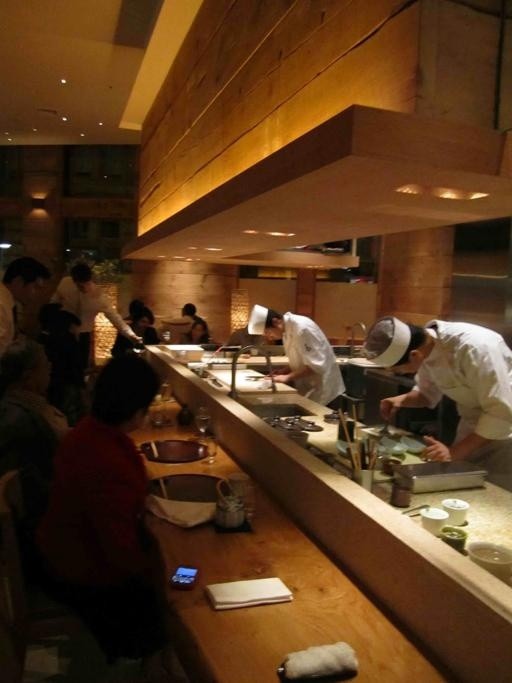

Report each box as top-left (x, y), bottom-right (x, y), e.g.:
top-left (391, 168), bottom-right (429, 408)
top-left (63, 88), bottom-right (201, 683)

top-left (347, 319), bottom-right (367, 358)
top-left (233, 345), bottom-right (277, 395)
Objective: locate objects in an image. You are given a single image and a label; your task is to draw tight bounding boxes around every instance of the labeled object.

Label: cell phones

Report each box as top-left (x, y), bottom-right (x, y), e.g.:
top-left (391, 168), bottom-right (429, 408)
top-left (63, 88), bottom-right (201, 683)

top-left (168, 564), bottom-right (200, 591)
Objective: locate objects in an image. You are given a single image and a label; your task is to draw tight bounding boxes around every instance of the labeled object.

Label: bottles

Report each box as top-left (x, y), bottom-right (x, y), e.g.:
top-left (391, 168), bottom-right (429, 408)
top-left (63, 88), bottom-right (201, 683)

top-left (388, 479), bottom-right (413, 508)
top-left (176, 402), bottom-right (193, 426)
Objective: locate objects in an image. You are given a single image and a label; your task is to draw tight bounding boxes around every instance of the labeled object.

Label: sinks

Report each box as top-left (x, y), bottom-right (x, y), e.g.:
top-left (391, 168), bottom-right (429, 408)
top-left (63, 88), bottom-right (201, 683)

top-left (242, 402), bottom-right (317, 421)
top-left (238, 394), bottom-right (294, 405)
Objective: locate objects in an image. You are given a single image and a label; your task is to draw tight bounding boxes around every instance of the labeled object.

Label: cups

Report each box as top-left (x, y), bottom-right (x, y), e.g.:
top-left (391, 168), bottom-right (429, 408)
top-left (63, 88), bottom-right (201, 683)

top-left (441, 498), bottom-right (469, 527)
top-left (438, 524), bottom-right (467, 553)
top-left (228, 472), bottom-right (258, 519)
top-left (351, 466), bottom-right (374, 493)
top-left (161, 383), bottom-right (171, 402)
top-left (204, 435), bottom-right (218, 463)
top-left (419, 506), bottom-right (448, 536)
top-left (337, 420), bottom-right (356, 443)
top-left (151, 410), bottom-right (165, 427)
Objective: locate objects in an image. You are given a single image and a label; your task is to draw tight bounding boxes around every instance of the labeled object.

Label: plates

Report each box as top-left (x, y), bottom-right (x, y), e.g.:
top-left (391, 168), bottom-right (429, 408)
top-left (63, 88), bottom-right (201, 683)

top-left (337, 424), bottom-right (427, 460)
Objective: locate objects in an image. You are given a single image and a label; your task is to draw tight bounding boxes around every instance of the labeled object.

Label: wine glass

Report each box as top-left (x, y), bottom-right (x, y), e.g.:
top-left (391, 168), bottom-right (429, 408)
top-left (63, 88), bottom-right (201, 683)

top-left (194, 406), bottom-right (212, 444)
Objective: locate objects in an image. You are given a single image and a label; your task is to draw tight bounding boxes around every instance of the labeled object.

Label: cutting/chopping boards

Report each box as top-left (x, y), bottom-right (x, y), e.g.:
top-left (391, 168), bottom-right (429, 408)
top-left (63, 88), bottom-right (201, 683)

top-left (206, 368), bottom-right (300, 394)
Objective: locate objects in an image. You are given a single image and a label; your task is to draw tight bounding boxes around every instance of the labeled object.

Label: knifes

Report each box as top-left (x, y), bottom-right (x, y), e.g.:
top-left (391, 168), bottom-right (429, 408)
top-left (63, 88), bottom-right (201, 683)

top-left (245, 374), bottom-right (276, 379)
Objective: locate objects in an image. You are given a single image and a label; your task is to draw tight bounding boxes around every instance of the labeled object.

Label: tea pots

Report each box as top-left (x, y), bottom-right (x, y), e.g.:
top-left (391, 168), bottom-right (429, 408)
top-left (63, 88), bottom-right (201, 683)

top-left (214, 477), bottom-right (247, 529)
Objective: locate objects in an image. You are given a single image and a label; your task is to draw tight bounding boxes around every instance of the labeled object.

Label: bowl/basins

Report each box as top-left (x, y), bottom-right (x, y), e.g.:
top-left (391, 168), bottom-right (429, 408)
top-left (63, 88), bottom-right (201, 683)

top-left (381, 458), bottom-right (402, 476)
top-left (468, 538), bottom-right (512, 582)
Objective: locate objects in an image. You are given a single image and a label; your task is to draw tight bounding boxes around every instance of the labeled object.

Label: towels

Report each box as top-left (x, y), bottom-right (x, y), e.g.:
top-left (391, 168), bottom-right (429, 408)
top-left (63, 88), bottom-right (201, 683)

top-left (204, 577), bottom-right (294, 611)
top-left (275, 640), bottom-right (358, 682)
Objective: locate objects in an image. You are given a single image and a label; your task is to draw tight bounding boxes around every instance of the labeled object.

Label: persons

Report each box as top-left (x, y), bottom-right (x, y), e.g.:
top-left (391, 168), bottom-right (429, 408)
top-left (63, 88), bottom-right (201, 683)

top-left (364, 315), bottom-right (512, 462)
top-left (248, 303), bottom-right (346, 410)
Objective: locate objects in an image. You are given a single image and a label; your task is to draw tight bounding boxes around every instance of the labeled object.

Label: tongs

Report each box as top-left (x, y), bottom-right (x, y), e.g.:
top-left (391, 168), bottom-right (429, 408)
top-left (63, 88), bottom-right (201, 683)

top-left (401, 503), bottom-right (431, 518)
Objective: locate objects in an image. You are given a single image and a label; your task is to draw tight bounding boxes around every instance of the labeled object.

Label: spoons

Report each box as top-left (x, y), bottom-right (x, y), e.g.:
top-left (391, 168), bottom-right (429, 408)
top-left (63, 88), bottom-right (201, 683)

top-left (379, 418), bottom-right (391, 435)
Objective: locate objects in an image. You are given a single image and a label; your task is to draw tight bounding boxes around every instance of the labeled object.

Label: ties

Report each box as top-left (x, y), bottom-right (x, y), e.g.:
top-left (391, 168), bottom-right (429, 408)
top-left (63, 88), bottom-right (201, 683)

top-left (74, 289), bottom-right (85, 342)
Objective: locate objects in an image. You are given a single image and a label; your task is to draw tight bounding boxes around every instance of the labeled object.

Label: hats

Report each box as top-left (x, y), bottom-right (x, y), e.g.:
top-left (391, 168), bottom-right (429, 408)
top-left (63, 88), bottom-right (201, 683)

top-left (362, 315), bottom-right (413, 369)
top-left (247, 304), bottom-right (269, 336)
